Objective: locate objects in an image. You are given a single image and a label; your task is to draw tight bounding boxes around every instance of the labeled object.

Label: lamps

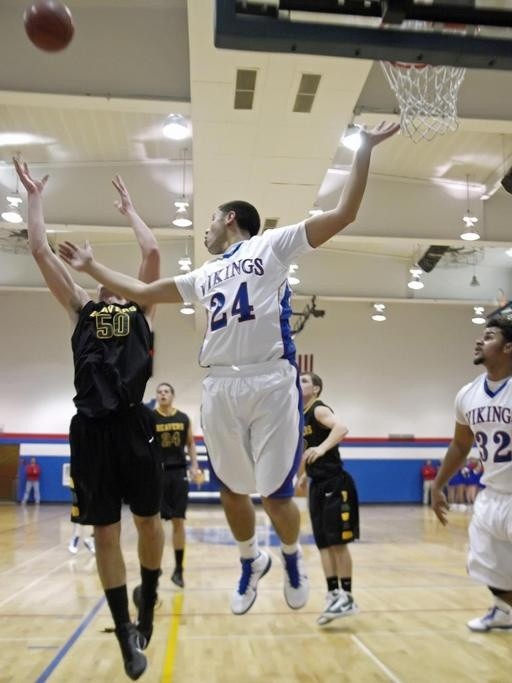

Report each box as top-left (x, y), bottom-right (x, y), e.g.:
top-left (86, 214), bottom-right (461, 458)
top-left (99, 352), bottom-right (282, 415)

top-left (179, 238), bottom-right (192, 273)
top-left (470, 247), bottom-right (479, 287)
top-left (459, 172), bottom-right (480, 242)
top-left (162, 113), bottom-right (189, 142)
top-left (408, 243), bottom-right (424, 291)
top-left (180, 303), bottom-right (195, 315)
top-left (173, 147), bottom-right (193, 227)
top-left (1, 148), bottom-right (24, 227)
top-left (342, 126), bottom-right (363, 152)
top-left (471, 306), bottom-right (487, 324)
top-left (372, 303), bottom-right (386, 322)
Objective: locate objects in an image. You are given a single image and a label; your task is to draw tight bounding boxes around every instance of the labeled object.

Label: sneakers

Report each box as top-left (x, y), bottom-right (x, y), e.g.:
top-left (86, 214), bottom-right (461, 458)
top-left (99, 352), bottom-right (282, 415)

top-left (68, 534), bottom-right (96, 554)
top-left (113, 622), bottom-right (148, 680)
top-left (171, 568), bottom-right (184, 587)
top-left (281, 546), bottom-right (307, 609)
top-left (132, 583), bottom-right (157, 642)
top-left (317, 589), bottom-right (357, 626)
top-left (232, 552), bottom-right (271, 615)
top-left (466, 604), bottom-right (512, 632)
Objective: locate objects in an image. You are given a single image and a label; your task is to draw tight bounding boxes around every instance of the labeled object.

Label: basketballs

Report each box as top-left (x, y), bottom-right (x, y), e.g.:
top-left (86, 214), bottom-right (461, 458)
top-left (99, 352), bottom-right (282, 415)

top-left (24, 0), bottom-right (72, 50)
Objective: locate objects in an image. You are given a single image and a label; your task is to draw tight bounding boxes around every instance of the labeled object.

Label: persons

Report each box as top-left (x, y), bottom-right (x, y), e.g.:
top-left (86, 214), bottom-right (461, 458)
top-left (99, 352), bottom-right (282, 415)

top-left (58, 120), bottom-right (401, 616)
top-left (20, 456), bottom-right (42, 506)
top-left (299, 371), bottom-right (363, 626)
top-left (67, 482), bottom-right (97, 553)
top-left (150, 383), bottom-right (200, 591)
top-left (429, 318), bottom-right (511, 632)
top-left (11, 156), bottom-right (165, 681)
top-left (422, 456), bottom-right (486, 506)
